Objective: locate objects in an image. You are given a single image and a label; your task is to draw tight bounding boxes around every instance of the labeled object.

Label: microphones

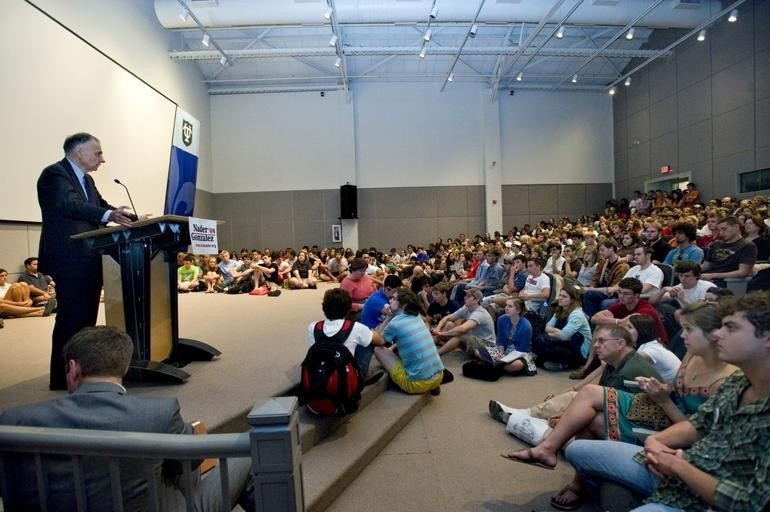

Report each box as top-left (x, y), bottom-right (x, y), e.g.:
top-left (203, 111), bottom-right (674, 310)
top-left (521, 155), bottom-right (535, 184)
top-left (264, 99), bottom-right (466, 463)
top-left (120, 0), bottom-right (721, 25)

top-left (114, 179), bottom-right (139, 220)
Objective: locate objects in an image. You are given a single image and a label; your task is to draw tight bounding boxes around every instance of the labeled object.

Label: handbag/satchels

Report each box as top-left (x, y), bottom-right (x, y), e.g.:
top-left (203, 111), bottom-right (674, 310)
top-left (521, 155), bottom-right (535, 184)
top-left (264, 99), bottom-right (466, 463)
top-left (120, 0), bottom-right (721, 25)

top-left (459, 358), bottom-right (503, 382)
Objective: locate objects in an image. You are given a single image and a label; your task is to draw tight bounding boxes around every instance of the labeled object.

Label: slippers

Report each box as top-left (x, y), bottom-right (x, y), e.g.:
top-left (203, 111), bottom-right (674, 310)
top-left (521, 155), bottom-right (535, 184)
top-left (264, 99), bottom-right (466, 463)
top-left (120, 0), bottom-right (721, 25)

top-left (500, 447), bottom-right (558, 472)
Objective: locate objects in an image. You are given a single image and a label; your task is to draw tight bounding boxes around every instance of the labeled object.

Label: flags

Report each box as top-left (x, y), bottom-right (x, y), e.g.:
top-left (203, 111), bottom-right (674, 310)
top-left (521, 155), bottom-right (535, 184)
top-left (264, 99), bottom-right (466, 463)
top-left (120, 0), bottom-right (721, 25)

top-left (186, 214), bottom-right (221, 258)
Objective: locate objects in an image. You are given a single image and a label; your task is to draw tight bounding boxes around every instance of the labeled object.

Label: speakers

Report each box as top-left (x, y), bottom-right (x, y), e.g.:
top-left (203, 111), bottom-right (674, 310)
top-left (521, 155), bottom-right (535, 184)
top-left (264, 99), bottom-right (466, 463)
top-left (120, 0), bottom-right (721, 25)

top-left (341, 185), bottom-right (357, 218)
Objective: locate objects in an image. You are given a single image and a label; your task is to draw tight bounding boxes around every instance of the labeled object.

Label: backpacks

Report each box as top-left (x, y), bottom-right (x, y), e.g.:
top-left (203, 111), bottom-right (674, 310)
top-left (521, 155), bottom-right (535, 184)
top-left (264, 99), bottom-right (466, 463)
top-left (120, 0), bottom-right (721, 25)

top-left (298, 318), bottom-right (362, 421)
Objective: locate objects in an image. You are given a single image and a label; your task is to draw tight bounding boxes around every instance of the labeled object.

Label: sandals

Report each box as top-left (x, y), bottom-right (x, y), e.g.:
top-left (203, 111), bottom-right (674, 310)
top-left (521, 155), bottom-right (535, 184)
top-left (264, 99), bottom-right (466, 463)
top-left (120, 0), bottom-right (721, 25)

top-left (551, 482), bottom-right (582, 511)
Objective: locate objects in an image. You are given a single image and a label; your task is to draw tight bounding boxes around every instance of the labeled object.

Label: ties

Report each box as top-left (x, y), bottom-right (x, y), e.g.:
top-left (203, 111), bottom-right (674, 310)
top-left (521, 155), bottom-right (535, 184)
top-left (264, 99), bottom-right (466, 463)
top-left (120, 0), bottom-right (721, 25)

top-left (82, 174), bottom-right (97, 206)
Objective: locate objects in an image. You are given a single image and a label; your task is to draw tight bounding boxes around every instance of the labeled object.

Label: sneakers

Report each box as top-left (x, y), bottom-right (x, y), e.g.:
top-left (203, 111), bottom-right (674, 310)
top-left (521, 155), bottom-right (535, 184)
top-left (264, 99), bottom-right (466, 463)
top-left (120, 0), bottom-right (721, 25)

top-left (569, 366), bottom-right (590, 380)
top-left (488, 398), bottom-right (523, 425)
top-left (207, 286), bottom-right (281, 297)
top-left (363, 367), bottom-right (384, 386)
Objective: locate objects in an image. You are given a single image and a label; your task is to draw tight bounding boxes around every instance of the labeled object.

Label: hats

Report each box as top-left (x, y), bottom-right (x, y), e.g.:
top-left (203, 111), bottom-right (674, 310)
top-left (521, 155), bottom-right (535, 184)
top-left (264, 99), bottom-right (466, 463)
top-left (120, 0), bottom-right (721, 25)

top-left (464, 287), bottom-right (484, 302)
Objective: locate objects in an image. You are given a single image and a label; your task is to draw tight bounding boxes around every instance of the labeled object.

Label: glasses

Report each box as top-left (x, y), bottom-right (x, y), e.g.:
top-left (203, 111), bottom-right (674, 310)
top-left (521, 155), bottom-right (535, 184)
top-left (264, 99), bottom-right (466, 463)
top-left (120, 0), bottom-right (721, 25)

top-left (594, 336), bottom-right (622, 345)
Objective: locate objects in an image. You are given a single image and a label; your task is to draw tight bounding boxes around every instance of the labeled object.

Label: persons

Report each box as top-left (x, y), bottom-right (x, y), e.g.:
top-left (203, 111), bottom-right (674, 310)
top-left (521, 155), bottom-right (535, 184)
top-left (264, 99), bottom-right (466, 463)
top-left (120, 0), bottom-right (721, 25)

top-left (0, 298), bottom-right (56, 319)
top-left (35, 131), bottom-right (155, 392)
top-left (1, 325), bottom-right (262, 511)
top-left (17, 257), bottom-right (59, 313)
top-left (0, 268), bottom-right (35, 308)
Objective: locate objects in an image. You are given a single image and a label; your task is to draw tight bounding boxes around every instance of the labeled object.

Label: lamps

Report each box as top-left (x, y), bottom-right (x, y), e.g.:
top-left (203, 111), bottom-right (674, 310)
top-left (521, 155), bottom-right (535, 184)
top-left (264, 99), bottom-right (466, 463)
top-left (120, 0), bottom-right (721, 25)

top-left (174, 2), bottom-right (232, 70)
top-left (569, 0), bottom-right (663, 82)
top-left (443, 0), bottom-right (491, 85)
top-left (517, 0), bottom-right (582, 82)
top-left (415, 0), bottom-right (442, 60)
top-left (320, 1), bottom-right (349, 72)
top-left (607, 1), bottom-right (744, 97)
